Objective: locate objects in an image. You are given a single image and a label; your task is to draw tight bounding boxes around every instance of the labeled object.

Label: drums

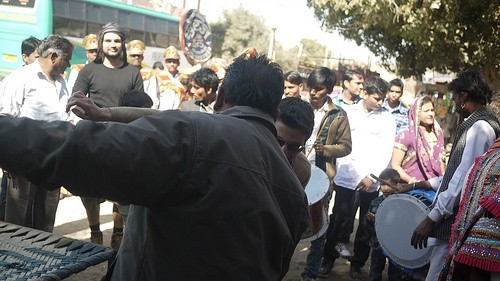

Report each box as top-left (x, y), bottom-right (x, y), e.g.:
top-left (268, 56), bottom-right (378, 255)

top-left (373, 189), bottom-right (440, 272)
top-left (298, 164), bottom-right (331, 242)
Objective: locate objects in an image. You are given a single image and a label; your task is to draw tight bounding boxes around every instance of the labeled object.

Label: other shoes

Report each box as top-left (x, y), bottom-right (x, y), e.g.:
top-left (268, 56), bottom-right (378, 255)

top-left (317, 257), bottom-right (335, 278)
top-left (369, 269), bottom-right (382, 281)
top-left (338, 242), bottom-right (351, 257)
top-left (300, 273), bottom-right (317, 281)
top-left (350, 262), bottom-right (362, 280)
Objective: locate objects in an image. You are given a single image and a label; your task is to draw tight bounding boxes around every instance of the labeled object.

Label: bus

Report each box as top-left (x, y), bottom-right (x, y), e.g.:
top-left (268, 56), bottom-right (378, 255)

top-left (0, 0), bottom-right (181, 85)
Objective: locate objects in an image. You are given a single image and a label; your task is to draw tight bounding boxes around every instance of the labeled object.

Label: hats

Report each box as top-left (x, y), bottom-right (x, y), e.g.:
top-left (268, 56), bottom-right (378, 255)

top-left (164, 46), bottom-right (180, 61)
top-left (128, 40), bottom-right (145, 55)
top-left (83, 34), bottom-right (99, 51)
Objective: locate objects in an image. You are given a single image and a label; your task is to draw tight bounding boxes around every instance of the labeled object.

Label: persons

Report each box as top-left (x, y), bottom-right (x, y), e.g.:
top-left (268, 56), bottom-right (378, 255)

top-left (64, 91), bottom-right (331, 281)
top-left (70, 24), bottom-right (145, 250)
top-left (180, 69), bottom-right (220, 114)
top-left (68, 33), bottom-right (100, 97)
top-left (127, 38), bottom-right (160, 110)
top-left (0, 48), bottom-right (312, 281)
top-left (298, 66), bottom-right (353, 281)
top-left (153, 61), bottom-right (177, 110)
top-left (21, 36), bottom-right (42, 67)
top-left (163, 44), bottom-right (189, 111)
top-left (283, 67), bottom-right (500, 281)
top-left (0, 35), bottom-right (76, 234)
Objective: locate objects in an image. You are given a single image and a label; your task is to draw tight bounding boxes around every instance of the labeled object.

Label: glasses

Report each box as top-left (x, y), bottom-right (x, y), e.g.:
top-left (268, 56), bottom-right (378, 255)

top-left (277, 138), bottom-right (306, 154)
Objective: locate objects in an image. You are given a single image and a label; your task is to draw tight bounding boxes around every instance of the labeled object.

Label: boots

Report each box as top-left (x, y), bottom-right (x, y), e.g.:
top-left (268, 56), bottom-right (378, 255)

top-left (111, 233), bottom-right (123, 251)
top-left (91, 231), bottom-right (103, 246)
